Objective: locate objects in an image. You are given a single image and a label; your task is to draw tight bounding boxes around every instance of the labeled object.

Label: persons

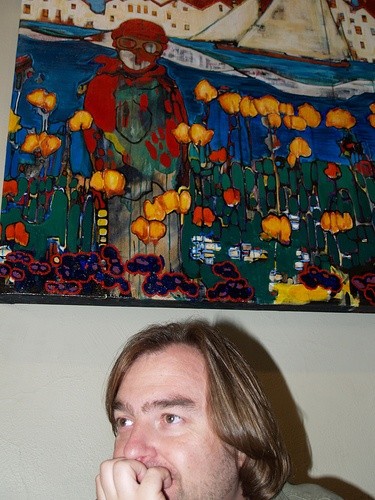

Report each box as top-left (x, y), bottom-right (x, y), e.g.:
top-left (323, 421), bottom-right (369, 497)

top-left (94, 318), bottom-right (341, 499)
